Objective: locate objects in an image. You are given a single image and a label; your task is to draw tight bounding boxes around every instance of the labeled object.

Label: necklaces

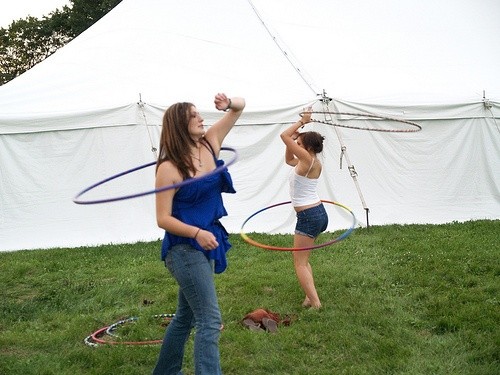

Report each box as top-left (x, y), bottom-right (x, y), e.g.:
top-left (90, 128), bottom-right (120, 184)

top-left (192, 147), bottom-right (203, 167)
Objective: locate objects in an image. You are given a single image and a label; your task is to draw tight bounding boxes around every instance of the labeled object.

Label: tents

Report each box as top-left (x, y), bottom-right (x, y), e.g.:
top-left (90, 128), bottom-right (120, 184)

top-left (0, 1), bottom-right (500, 251)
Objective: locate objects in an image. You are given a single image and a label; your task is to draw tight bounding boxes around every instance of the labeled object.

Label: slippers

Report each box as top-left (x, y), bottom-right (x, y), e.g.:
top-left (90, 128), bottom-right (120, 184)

top-left (243, 318), bottom-right (266, 335)
top-left (262, 317), bottom-right (279, 334)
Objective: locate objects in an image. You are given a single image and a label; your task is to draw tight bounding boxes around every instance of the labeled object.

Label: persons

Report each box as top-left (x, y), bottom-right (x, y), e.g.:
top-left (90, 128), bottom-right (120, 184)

top-left (280, 106), bottom-right (329, 314)
top-left (151, 93), bottom-right (245, 375)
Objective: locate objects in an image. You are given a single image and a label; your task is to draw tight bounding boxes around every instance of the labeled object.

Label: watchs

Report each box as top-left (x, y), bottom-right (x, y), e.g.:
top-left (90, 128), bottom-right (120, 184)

top-left (223, 98), bottom-right (232, 112)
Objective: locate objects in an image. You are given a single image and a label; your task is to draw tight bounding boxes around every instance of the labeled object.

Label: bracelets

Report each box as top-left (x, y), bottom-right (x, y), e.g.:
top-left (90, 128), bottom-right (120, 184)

top-left (298, 120), bottom-right (304, 129)
top-left (194, 228), bottom-right (201, 240)
